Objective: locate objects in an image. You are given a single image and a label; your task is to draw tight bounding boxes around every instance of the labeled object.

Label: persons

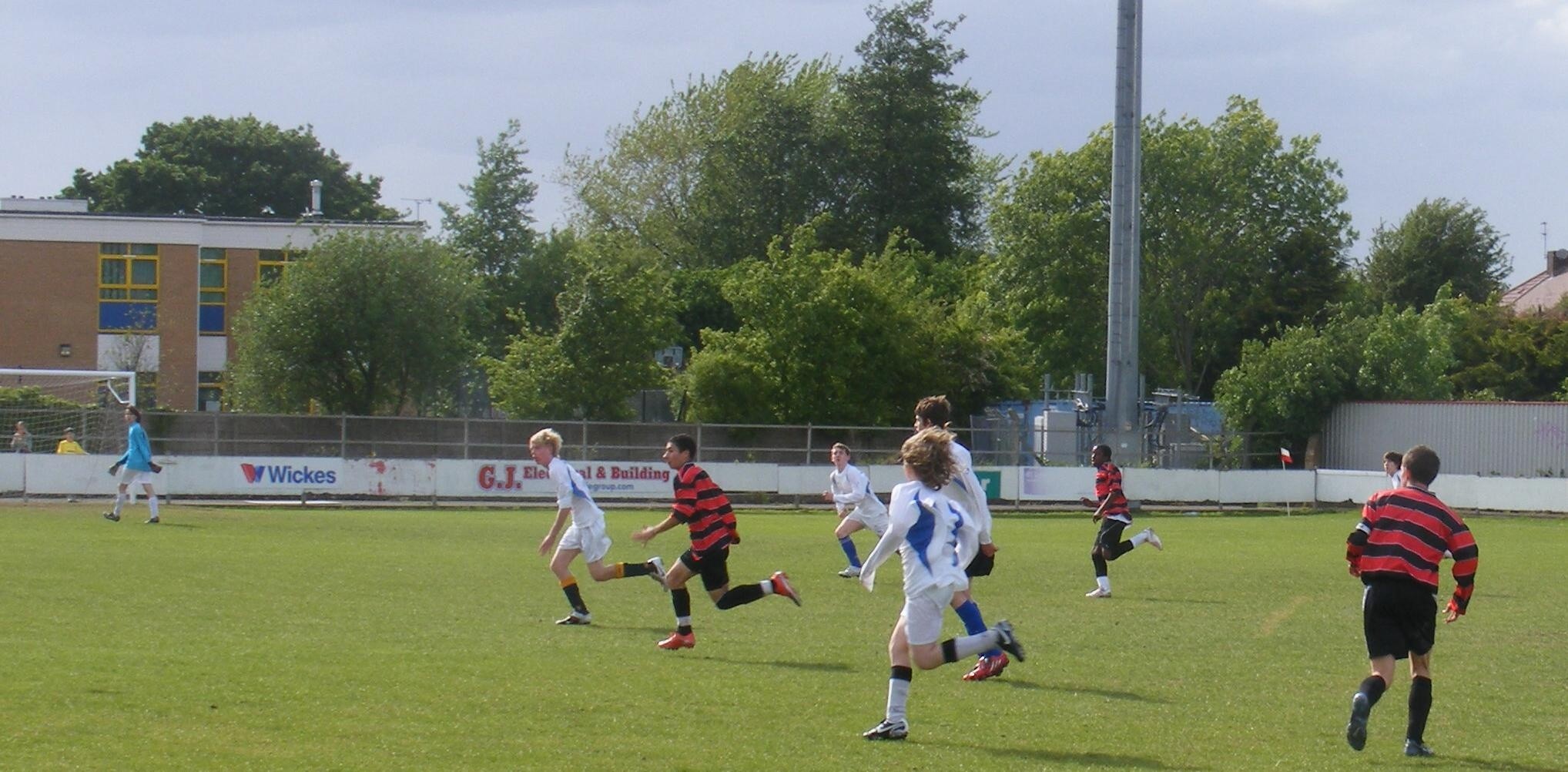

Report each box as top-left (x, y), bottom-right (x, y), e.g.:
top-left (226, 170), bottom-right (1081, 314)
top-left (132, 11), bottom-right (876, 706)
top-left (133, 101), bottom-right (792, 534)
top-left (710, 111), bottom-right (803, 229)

top-left (1346, 445), bottom-right (1479, 757)
top-left (103, 406), bottom-right (161, 523)
top-left (529, 429), bottom-right (668, 624)
top-left (631, 432), bottom-right (801, 650)
top-left (823, 442), bottom-right (902, 579)
top-left (1079, 444), bottom-right (1163, 598)
top-left (10, 421), bottom-right (32, 453)
top-left (1383, 450), bottom-right (1451, 559)
top-left (914, 396), bottom-right (1010, 681)
top-left (861, 426), bottom-right (1025, 741)
top-left (57, 427), bottom-right (90, 502)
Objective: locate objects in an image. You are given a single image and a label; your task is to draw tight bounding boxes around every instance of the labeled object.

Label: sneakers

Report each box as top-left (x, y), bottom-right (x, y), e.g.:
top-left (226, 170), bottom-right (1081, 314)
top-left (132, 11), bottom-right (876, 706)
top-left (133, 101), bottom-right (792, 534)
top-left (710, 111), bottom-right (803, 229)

top-left (840, 565), bottom-right (863, 578)
top-left (144, 517), bottom-right (159, 524)
top-left (864, 719), bottom-right (909, 740)
top-left (1346, 692), bottom-right (1368, 751)
top-left (964, 652), bottom-right (1010, 682)
top-left (555, 611), bottom-right (590, 626)
top-left (658, 631), bottom-right (696, 649)
top-left (1404, 740), bottom-right (1433, 757)
top-left (103, 511), bottom-right (120, 522)
top-left (646, 556), bottom-right (669, 592)
top-left (1142, 527), bottom-right (1163, 551)
top-left (1085, 588), bottom-right (1112, 598)
top-left (990, 620), bottom-right (1024, 662)
top-left (770, 571), bottom-right (801, 607)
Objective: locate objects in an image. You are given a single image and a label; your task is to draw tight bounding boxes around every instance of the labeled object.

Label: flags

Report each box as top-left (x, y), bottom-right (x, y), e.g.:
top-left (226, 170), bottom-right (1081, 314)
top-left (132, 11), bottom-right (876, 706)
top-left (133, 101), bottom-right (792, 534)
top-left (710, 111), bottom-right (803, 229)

top-left (1281, 448), bottom-right (1292, 463)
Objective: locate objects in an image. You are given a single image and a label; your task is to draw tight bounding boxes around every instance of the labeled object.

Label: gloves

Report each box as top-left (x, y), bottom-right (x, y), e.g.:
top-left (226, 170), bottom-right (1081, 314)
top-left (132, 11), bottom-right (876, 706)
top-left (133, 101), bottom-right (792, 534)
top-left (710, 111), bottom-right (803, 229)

top-left (148, 462), bottom-right (162, 473)
top-left (109, 462), bottom-right (119, 476)
